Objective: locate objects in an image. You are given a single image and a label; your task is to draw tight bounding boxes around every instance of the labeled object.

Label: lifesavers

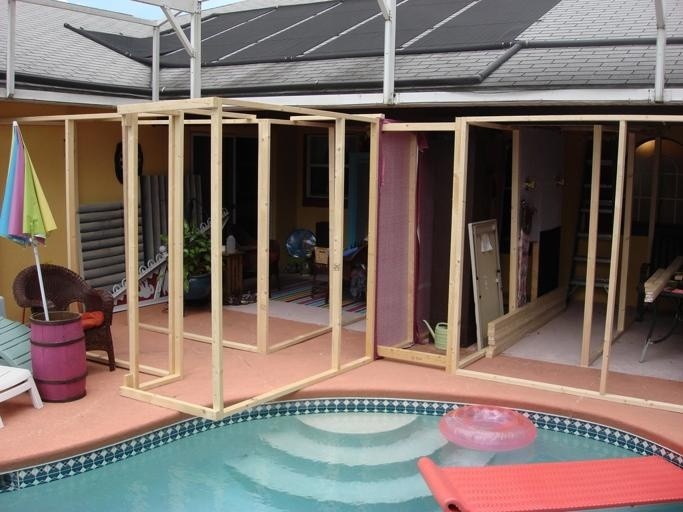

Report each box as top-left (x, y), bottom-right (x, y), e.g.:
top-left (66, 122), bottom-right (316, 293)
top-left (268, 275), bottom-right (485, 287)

top-left (439, 405), bottom-right (535, 450)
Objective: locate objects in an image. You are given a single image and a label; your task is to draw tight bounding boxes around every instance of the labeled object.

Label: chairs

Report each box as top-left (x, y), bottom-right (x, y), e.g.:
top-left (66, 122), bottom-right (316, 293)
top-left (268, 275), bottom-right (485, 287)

top-left (1, 362), bottom-right (46, 430)
top-left (12, 263), bottom-right (117, 373)
top-left (1, 296), bottom-right (35, 376)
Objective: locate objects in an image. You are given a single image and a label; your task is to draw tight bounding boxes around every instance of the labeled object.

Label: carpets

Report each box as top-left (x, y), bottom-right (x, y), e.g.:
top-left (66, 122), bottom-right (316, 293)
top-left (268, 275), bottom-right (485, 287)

top-left (266, 277), bottom-right (367, 316)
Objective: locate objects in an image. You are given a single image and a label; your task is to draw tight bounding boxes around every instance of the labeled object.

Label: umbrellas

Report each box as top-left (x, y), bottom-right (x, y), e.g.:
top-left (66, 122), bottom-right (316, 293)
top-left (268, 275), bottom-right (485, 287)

top-left (0, 120), bottom-right (59, 322)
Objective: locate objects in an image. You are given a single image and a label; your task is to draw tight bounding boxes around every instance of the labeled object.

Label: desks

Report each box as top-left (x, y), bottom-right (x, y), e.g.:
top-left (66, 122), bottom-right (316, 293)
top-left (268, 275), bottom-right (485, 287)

top-left (634, 274), bottom-right (683, 364)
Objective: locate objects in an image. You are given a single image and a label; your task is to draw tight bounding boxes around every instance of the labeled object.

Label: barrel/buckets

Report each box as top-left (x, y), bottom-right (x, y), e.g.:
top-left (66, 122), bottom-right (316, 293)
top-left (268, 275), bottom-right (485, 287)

top-left (28, 310), bottom-right (89, 403)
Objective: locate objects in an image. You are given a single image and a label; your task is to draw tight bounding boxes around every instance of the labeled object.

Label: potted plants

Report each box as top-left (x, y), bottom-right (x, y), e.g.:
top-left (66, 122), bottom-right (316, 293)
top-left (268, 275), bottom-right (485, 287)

top-left (159, 216), bottom-right (230, 303)
